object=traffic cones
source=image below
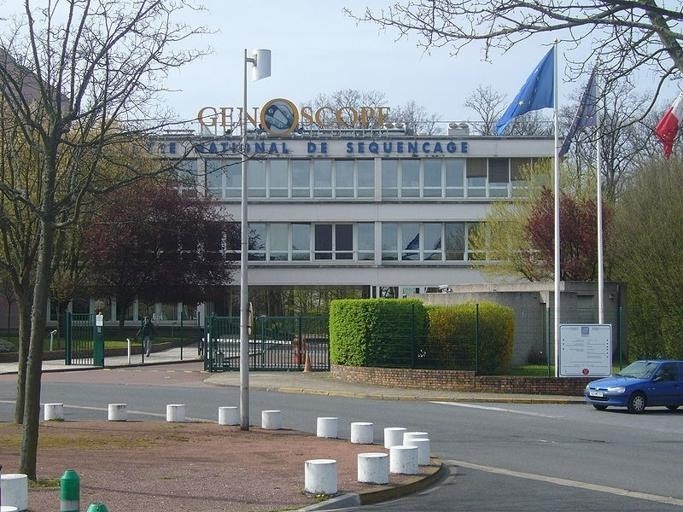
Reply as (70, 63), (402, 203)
(302, 351), (313, 373)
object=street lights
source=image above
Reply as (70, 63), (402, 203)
(240, 48), (272, 430)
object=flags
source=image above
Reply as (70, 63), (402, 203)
(431, 238), (442, 256)
(654, 91), (683, 160)
(493, 44), (555, 135)
(558, 66), (596, 161)
(406, 233), (420, 256)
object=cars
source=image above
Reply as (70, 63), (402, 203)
(585, 359), (683, 414)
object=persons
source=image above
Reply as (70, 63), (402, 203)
(137, 315), (154, 357)
